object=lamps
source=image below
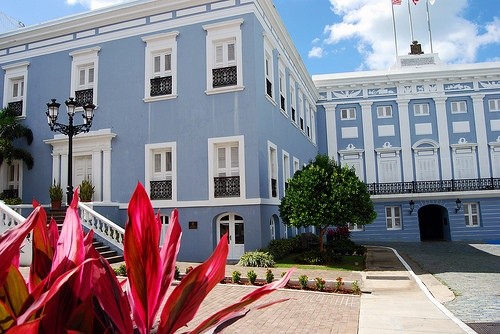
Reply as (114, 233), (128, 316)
(410, 201), (414, 215)
(455, 199), (461, 213)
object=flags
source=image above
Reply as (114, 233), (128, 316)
(412, 0), (421, 5)
(391, 0), (402, 5)
(429, 0), (436, 6)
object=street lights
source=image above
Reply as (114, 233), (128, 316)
(44, 95), (95, 209)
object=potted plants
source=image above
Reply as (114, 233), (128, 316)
(78, 181), (95, 202)
(50, 179), (63, 209)
